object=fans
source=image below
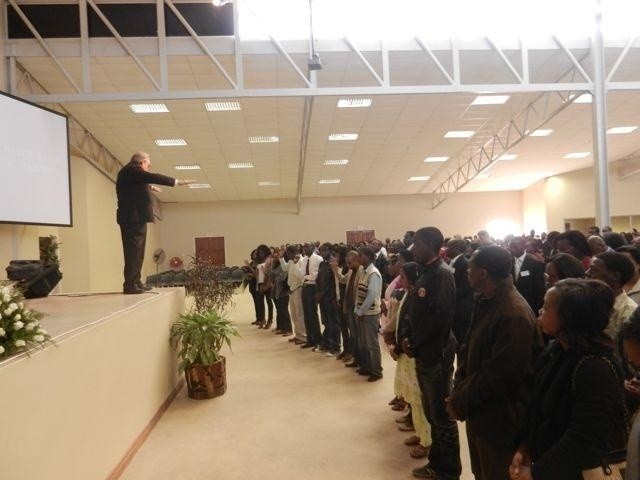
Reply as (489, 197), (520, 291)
(152, 249), (165, 287)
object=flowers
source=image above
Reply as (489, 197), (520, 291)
(0, 279), (60, 359)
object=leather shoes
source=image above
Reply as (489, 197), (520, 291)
(123, 283), (144, 294)
(326, 351), (341, 357)
(138, 280), (153, 291)
(312, 346), (317, 352)
(251, 320), (258, 325)
(283, 332), (293, 337)
(368, 374), (383, 382)
(315, 344), (327, 353)
(359, 367), (371, 375)
(288, 337), (298, 342)
(256, 320), (262, 325)
(346, 360), (360, 367)
(276, 330), (287, 334)
(295, 339), (306, 344)
(301, 343), (313, 350)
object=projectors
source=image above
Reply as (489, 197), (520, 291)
(308, 58), (323, 70)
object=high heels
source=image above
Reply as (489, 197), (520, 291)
(341, 354), (349, 360)
(264, 319), (273, 329)
(258, 319), (267, 329)
(343, 353), (353, 363)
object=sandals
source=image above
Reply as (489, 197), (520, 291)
(391, 397), (407, 411)
(389, 395), (400, 405)
(398, 421), (415, 431)
(410, 445), (427, 459)
(396, 413), (412, 423)
(404, 435), (420, 445)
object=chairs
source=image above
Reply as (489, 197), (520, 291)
(231, 270), (245, 288)
(147, 276), (158, 287)
(216, 270), (230, 289)
(159, 275), (171, 287)
(160, 266), (243, 275)
(171, 274), (188, 288)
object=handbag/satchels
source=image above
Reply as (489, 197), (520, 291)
(572, 355), (631, 480)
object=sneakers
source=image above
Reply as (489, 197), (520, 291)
(413, 462), (438, 477)
(335, 351), (346, 360)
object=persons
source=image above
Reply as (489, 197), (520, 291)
(603, 225), (612, 233)
(605, 231), (625, 247)
(398, 228), (463, 480)
(583, 253), (636, 340)
(279, 245), (307, 346)
(529, 230), (538, 241)
(269, 249), (294, 337)
(551, 230), (595, 270)
(244, 249), (267, 329)
(472, 236), (480, 244)
(539, 233), (547, 245)
(322, 244), (349, 353)
(543, 253), (585, 287)
(620, 232), (634, 240)
(315, 242), (335, 352)
(587, 235), (615, 253)
(445, 238), (472, 339)
(358, 241), (366, 250)
(248, 246), (278, 331)
(477, 230), (496, 246)
(447, 245), (545, 479)
(353, 246), (383, 381)
(295, 242), (324, 348)
(370, 238), (388, 299)
(618, 304), (639, 479)
(618, 242), (640, 261)
(510, 277), (627, 480)
(588, 226), (600, 236)
(386, 262), (432, 459)
(508, 236), (544, 295)
(115, 152), (196, 295)
(330, 250), (364, 367)
(548, 231), (561, 260)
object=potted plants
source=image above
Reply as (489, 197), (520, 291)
(169, 307), (244, 400)
